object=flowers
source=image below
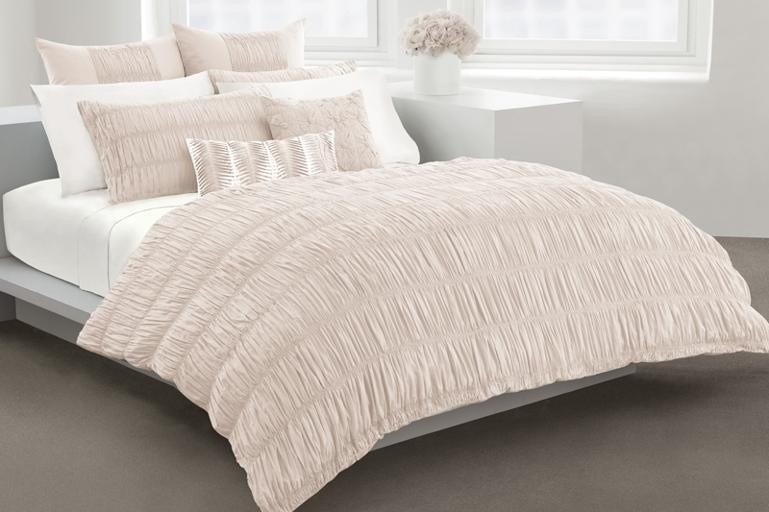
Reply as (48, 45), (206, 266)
(399, 9), (479, 60)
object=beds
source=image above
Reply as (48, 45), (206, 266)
(1, 16), (769, 512)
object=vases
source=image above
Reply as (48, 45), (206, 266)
(414, 52), (464, 97)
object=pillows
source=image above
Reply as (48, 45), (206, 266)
(26, 69), (218, 203)
(213, 68), (422, 175)
(170, 15), (312, 76)
(30, 28), (184, 85)
(183, 129), (342, 200)
(208, 56), (362, 91)
(74, 85), (275, 204)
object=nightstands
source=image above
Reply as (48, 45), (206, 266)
(389, 77), (584, 180)
